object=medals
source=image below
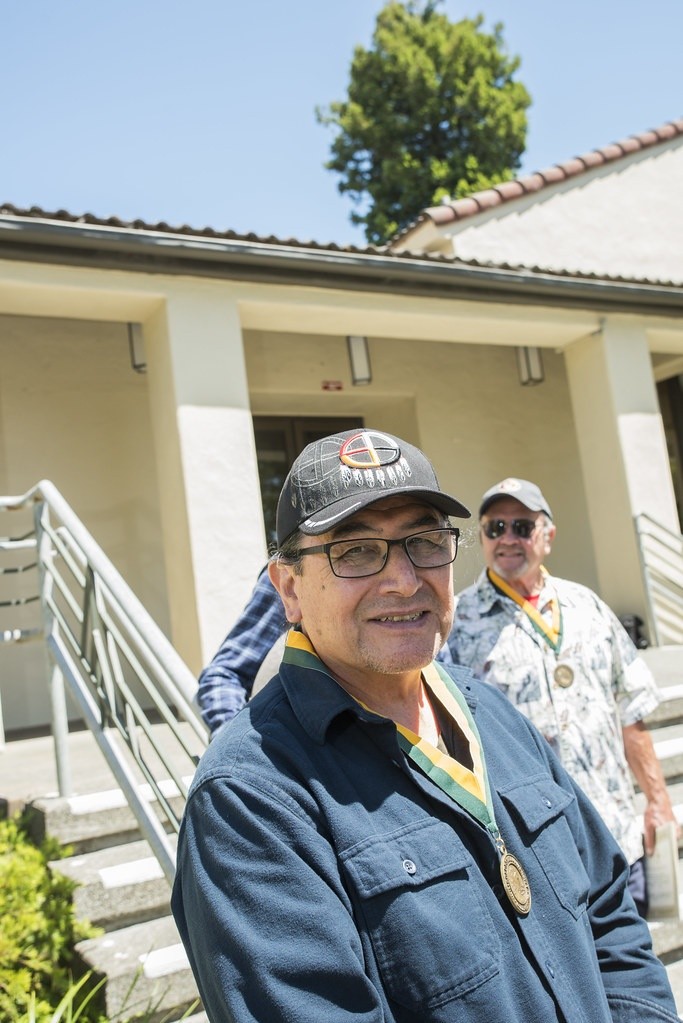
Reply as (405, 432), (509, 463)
(500, 853), (532, 914)
(554, 664), (575, 687)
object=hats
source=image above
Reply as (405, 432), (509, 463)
(276, 428), (471, 550)
(478, 478), (553, 522)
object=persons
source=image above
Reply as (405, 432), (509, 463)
(172, 430), (683, 1021)
(195, 557), (294, 731)
(449, 478), (683, 921)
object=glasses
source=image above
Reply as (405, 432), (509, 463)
(282, 528), (461, 578)
(481, 520), (545, 539)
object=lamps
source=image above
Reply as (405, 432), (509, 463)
(516, 349), (545, 384)
(346, 336), (371, 385)
(128, 322), (147, 373)
